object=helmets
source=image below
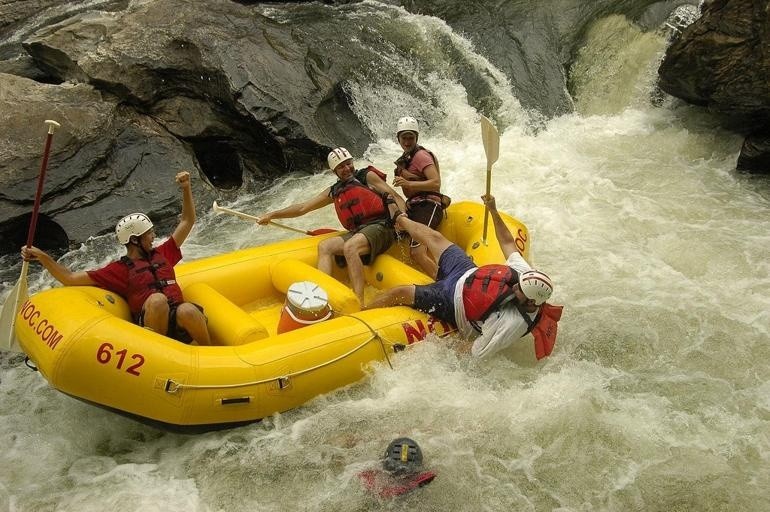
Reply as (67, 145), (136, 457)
(397, 117), (419, 137)
(328, 147), (353, 172)
(520, 269), (553, 306)
(383, 438), (423, 473)
(115, 213), (154, 245)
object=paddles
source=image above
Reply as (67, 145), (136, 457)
(211, 200), (340, 236)
(479, 112), (501, 248)
(0, 118), (62, 354)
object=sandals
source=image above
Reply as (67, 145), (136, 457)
(382, 192), (402, 229)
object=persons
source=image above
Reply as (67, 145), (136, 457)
(20, 170), (212, 348)
(356, 434), (438, 503)
(392, 116), (444, 281)
(256, 147), (410, 315)
(361, 189), (553, 363)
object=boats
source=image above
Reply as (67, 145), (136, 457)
(17, 200), (539, 431)
